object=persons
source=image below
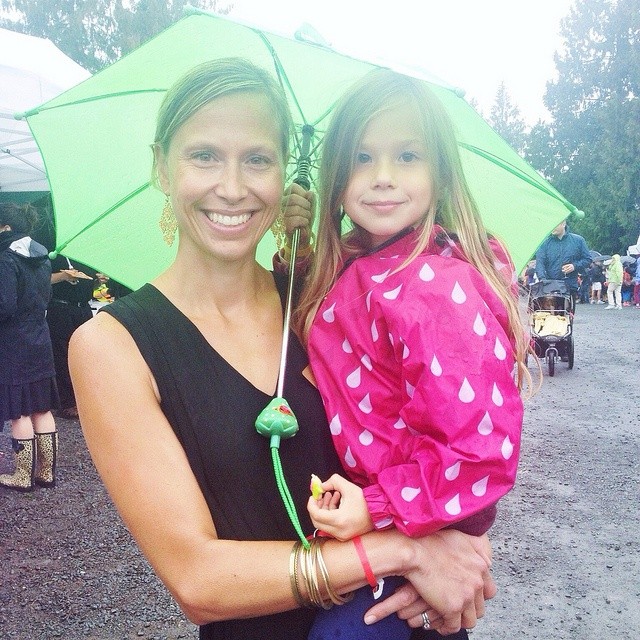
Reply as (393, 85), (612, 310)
(293, 69), (525, 640)
(66, 59), (498, 640)
(590, 248), (639, 309)
(49, 253), (98, 419)
(534, 218), (589, 315)
(1, 201), (59, 491)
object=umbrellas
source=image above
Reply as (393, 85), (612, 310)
(11, 4), (589, 440)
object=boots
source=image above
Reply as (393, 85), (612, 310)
(34, 431), (58, 489)
(1, 438), (36, 490)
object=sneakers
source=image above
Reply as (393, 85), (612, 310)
(603, 304), (614, 311)
(614, 304), (622, 309)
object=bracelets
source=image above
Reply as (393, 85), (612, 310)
(288, 537), (356, 613)
(350, 536), (378, 587)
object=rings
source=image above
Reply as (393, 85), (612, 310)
(421, 612), (433, 628)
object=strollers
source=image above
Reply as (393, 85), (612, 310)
(525, 269), (576, 378)
